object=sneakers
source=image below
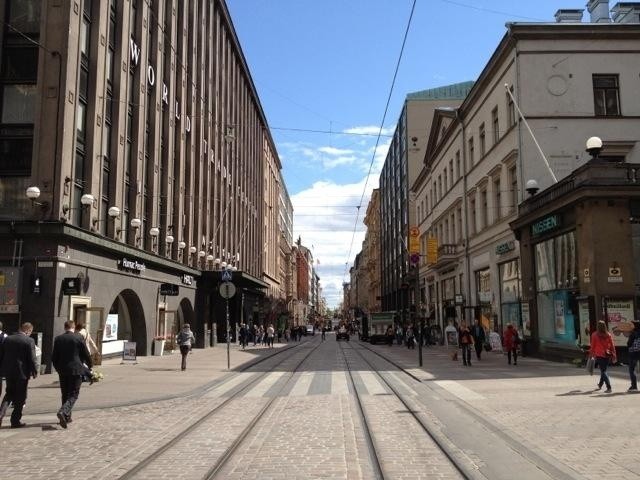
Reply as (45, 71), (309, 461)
(628, 387), (637, 391)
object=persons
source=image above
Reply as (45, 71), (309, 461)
(589, 320), (617, 394)
(1, 320), (99, 429)
(626, 321), (639, 391)
(239, 316), (522, 366)
(176, 325), (196, 370)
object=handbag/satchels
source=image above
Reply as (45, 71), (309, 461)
(511, 335), (521, 343)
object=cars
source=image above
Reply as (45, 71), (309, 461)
(298, 315), (350, 341)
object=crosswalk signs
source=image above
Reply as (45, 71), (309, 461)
(222, 270), (232, 280)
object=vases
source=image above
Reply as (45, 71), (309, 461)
(154, 340), (166, 356)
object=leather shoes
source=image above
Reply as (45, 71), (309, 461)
(11, 423), (25, 428)
(57, 411), (67, 428)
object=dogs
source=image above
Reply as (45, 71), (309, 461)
(448, 350), (459, 361)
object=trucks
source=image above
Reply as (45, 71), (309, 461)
(356, 309), (396, 345)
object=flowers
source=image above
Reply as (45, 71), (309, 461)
(154, 336), (165, 340)
(90, 371), (104, 381)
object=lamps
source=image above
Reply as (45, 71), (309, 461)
(92, 206), (120, 226)
(152, 235), (174, 250)
(223, 123), (238, 145)
(525, 180), (539, 197)
(408, 136), (421, 154)
(116, 218), (141, 235)
(137, 228), (159, 244)
(63, 194), (94, 215)
(585, 136), (604, 159)
(26, 185), (51, 212)
(169, 241), (237, 272)
